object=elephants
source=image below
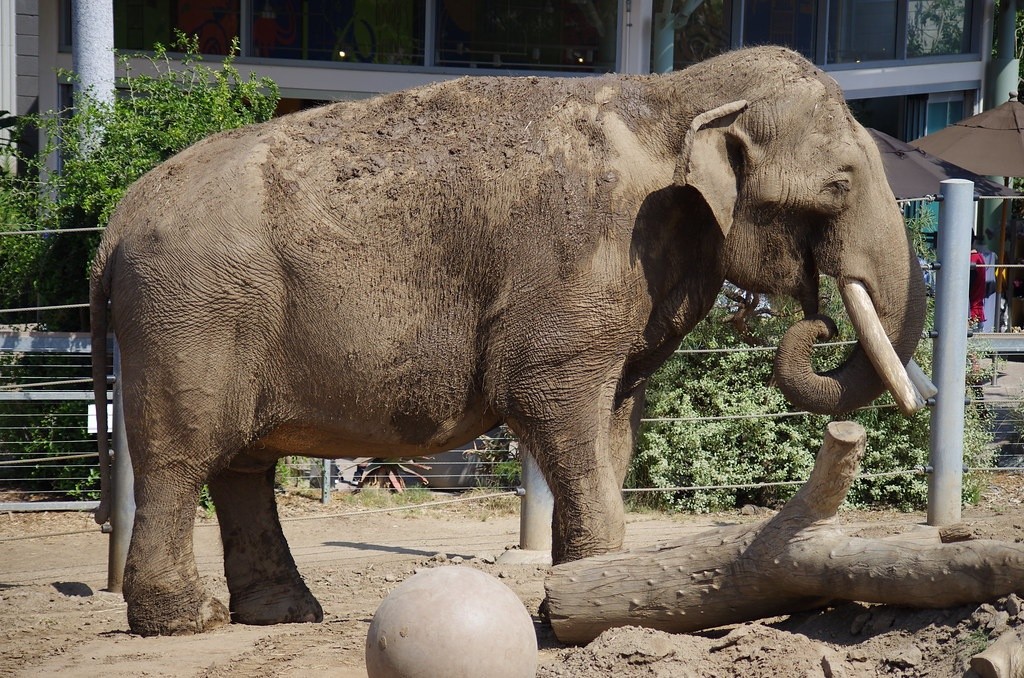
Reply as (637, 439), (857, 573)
(88, 43), (938, 639)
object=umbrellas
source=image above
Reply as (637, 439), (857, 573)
(865, 91), (1024, 388)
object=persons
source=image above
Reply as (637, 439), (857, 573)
(970, 242), (999, 334)
(967, 226), (987, 385)
